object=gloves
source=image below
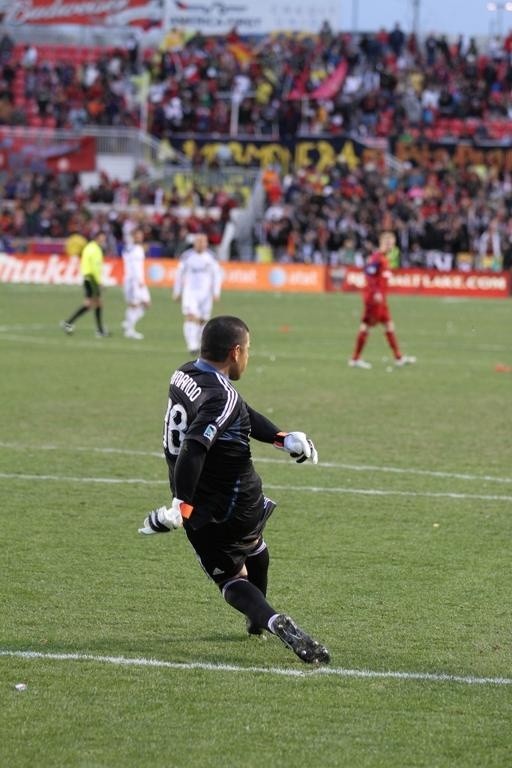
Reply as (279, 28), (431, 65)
(137, 498), (184, 535)
(273, 431), (319, 464)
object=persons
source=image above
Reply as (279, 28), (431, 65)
(58, 231), (110, 336)
(0, 15), (512, 275)
(138, 315), (333, 665)
(120, 230), (151, 341)
(172, 234), (224, 355)
(348, 232), (415, 369)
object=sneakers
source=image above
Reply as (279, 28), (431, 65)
(393, 355), (416, 367)
(267, 613), (331, 667)
(348, 358), (369, 370)
(59, 319), (144, 340)
(245, 618), (264, 638)
